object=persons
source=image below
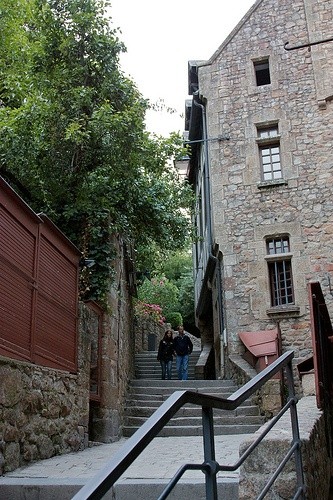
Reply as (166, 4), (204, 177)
(157, 330), (175, 380)
(171, 326), (193, 380)
